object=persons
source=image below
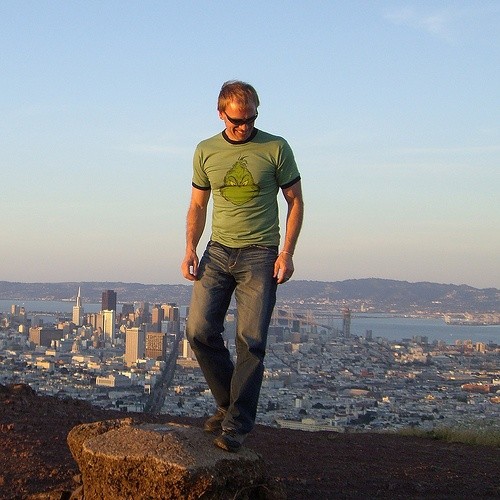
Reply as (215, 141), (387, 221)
(182, 80), (305, 454)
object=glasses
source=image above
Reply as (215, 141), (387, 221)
(224, 111), (258, 125)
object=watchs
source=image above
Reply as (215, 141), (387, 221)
(282, 250), (293, 257)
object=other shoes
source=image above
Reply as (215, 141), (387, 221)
(203, 407), (228, 433)
(214, 425), (250, 451)
